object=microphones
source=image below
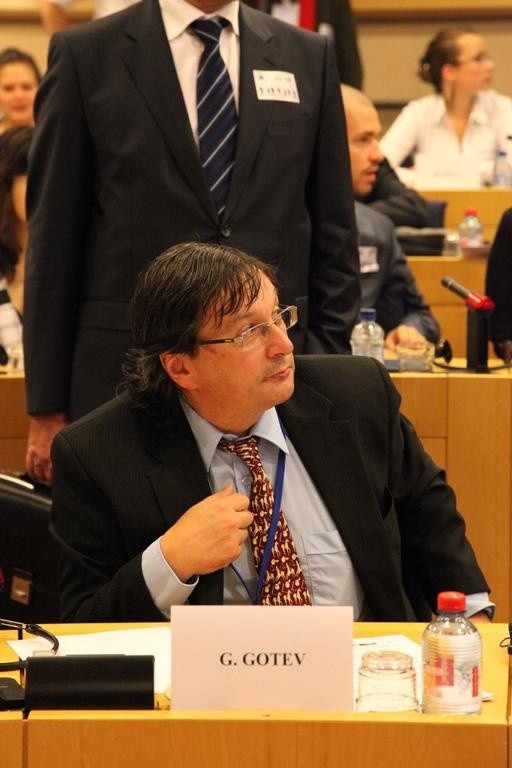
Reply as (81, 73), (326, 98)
(441, 276), (493, 311)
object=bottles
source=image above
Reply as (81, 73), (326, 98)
(460, 209), (483, 248)
(349, 307), (383, 367)
(422, 592), (483, 718)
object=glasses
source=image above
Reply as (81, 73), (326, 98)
(195, 305), (298, 351)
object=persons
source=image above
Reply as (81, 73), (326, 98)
(0, 48), (43, 373)
(23, 0), (362, 488)
(28, 240), (499, 625)
(486, 208), (511, 369)
(340, 82), (454, 365)
(372, 27), (510, 189)
(246, 0), (362, 92)
(42, 2), (136, 32)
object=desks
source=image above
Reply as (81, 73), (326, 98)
(2, 618), (511, 768)
(1, 357), (512, 624)
(0, 185), (510, 358)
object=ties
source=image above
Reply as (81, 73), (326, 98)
(190, 16), (239, 218)
(219, 435), (308, 606)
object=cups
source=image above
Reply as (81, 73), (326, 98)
(356, 650), (420, 712)
(396, 342), (436, 374)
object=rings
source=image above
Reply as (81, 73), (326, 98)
(32, 462), (45, 470)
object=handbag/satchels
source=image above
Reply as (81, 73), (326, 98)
(0, 472), (71, 628)
